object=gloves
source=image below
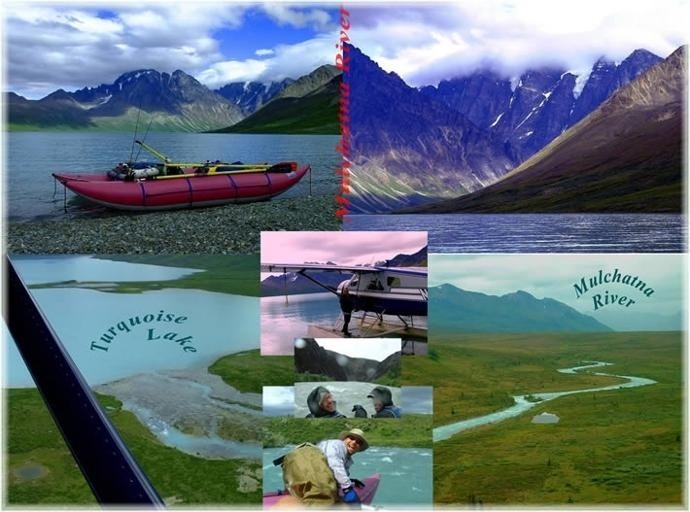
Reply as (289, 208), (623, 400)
(343, 488), (361, 504)
(350, 479), (364, 488)
(340, 429), (368, 451)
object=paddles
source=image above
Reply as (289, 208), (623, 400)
(152, 161), (297, 179)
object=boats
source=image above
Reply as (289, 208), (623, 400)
(263, 472), (382, 509)
(50, 160), (312, 212)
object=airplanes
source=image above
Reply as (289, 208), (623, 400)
(260, 262), (428, 356)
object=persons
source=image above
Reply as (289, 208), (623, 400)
(336, 273), (359, 336)
(274, 428), (369, 509)
(305, 386), (401, 419)
